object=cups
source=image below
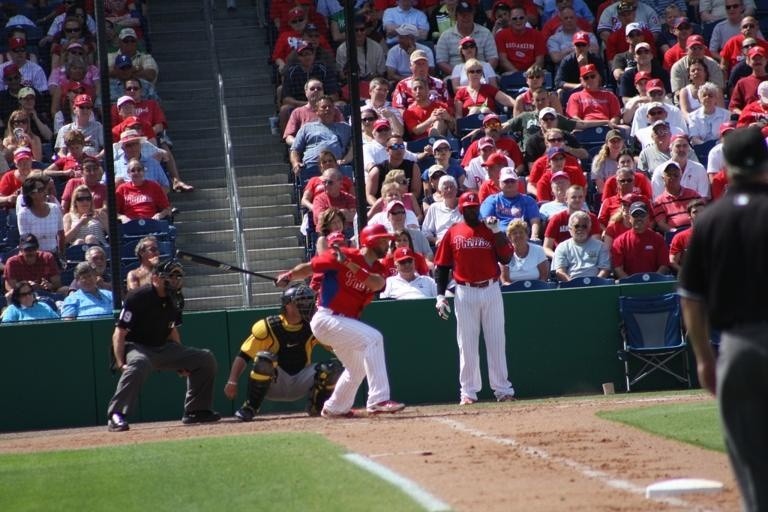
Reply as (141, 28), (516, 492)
(602, 383), (614, 394)
(13, 127), (26, 147)
(269, 117), (280, 136)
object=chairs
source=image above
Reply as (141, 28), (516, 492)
(618, 293), (692, 390)
(0, 1), (186, 316)
(294, 9), (727, 292)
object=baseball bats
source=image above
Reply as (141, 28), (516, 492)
(176, 248), (290, 286)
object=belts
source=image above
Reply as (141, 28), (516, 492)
(457, 278), (497, 287)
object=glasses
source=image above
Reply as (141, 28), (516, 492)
(10, 26), (143, 298)
(321, 180), (339, 185)
(511, 16), (526, 21)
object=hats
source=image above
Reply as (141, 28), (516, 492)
(456, 2), (473, 13)
(288, 7), (304, 22)
(395, 247), (413, 261)
(304, 24), (318, 34)
(617, 1), (636, 13)
(371, 32), (768, 216)
(395, 23), (419, 36)
(674, 17), (690, 29)
(296, 42), (313, 53)
(625, 23), (643, 38)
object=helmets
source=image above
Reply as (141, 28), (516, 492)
(282, 283), (315, 322)
(360, 225), (397, 251)
(155, 261), (185, 294)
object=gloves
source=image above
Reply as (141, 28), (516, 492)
(273, 273), (292, 286)
(436, 295), (452, 320)
(328, 244), (346, 263)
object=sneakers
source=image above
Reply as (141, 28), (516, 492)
(308, 402), (325, 417)
(365, 400), (405, 415)
(459, 397), (474, 407)
(107, 413), (128, 432)
(321, 405), (363, 417)
(235, 400), (260, 422)
(496, 393), (514, 402)
(182, 411), (222, 424)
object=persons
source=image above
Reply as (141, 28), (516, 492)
(677, 127), (766, 512)
(431, 188), (518, 407)
(228, 2), (768, 306)
(1, 1), (194, 322)
(224, 285), (343, 420)
(269, 223), (408, 420)
(105, 260), (220, 433)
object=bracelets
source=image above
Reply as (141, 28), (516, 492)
(227, 381), (239, 387)
